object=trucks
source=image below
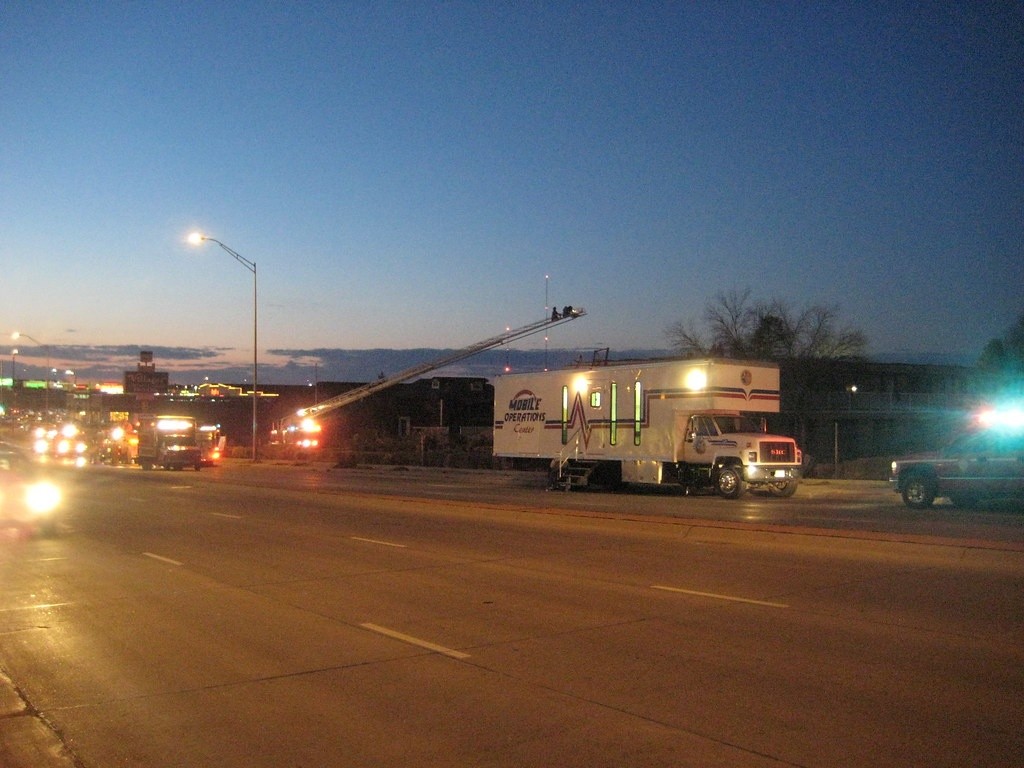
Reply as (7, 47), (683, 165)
(135, 413), (203, 473)
(494, 357), (806, 499)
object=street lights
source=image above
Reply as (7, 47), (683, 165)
(187, 234), (258, 466)
(12, 333), (49, 423)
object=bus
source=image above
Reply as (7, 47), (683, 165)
(197, 425), (222, 467)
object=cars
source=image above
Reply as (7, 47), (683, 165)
(888, 421), (1023, 510)
